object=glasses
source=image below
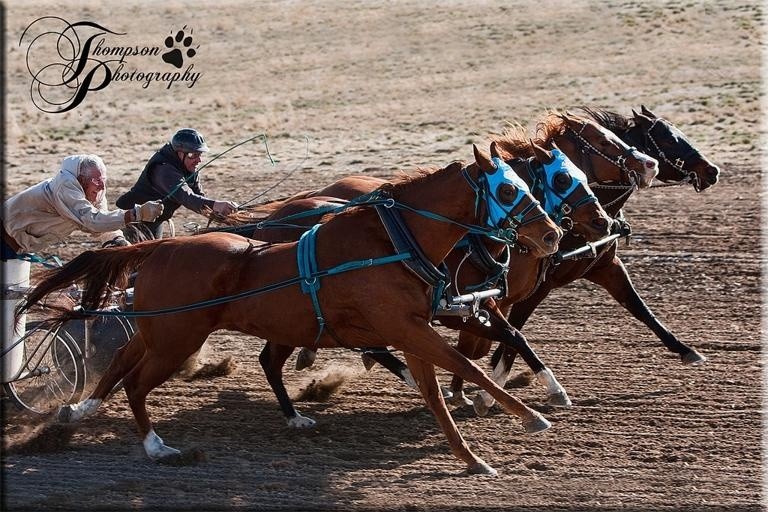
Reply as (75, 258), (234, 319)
(188, 152), (200, 159)
(79, 175), (106, 186)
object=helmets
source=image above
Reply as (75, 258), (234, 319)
(170, 128), (209, 152)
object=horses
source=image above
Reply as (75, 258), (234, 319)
(13, 101), (723, 478)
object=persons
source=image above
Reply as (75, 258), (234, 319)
(116, 128), (238, 239)
(3, 154), (164, 259)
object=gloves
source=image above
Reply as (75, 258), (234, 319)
(134, 199), (164, 223)
